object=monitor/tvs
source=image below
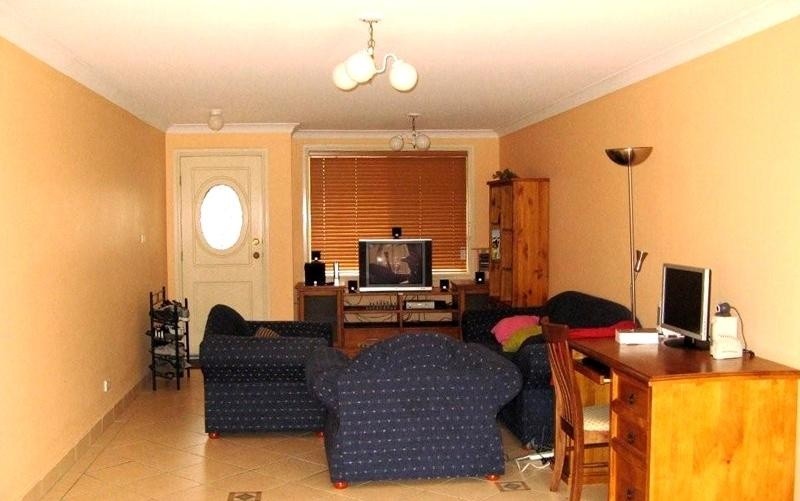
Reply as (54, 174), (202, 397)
(661, 264), (711, 341)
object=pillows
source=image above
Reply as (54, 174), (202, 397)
(253, 326), (280, 337)
(490, 313), (539, 341)
(501, 324), (543, 351)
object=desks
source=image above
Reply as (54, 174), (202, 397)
(550, 329), (800, 500)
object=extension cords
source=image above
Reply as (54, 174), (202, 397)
(528, 451), (555, 460)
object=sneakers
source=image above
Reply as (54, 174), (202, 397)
(149, 300), (190, 323)
(149, 343), (187, 357)
(146, 324), (184, 341)
(149, 359), (192, 378)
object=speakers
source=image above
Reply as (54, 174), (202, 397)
(440, 279), (449, 292)
(392, 227), (402, 239)
(311, 250), (320, 263)
(475, 272), (485, 284)
(304, 263), (325, 286)
(348, 280), (357, 293)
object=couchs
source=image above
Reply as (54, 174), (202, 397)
(461, 288), (643, 449)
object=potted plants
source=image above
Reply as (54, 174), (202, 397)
(491, 167), (519, 180)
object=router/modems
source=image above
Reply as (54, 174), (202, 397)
(661, 326), (685, 339)
(406, 300), (435, 309)
(614, 328), (658, 345)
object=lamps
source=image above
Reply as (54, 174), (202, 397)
(605, 144), (657, 344)
(388, 111), (431, 152)
(331, 13), (418, 92)
(206, 108), (224, 131)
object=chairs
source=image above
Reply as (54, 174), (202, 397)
(307, 330), (527, 489)
(539, 322), (610, 500)
(198, 301), (333, 439)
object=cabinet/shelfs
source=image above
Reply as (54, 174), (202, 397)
(293, 280), (488, 358)
(148, 285), (191, 392)
(486, 177), (550, 307)
